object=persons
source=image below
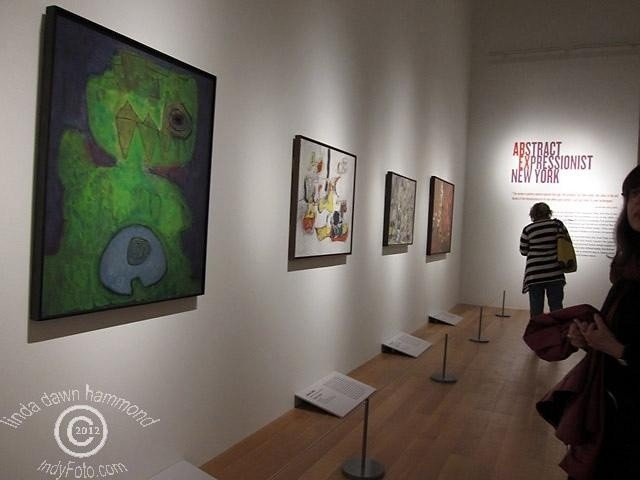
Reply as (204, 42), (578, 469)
(567, 166), (639, 479)
(519, 202), (572, 318)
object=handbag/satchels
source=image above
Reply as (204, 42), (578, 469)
(556, 218), (577, 273)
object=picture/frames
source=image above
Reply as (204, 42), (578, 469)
(382, 169), (417, 246)
(29, 2), (218, 326)
(289, 133), (357, 261)
(424, 174), (456, 257)
